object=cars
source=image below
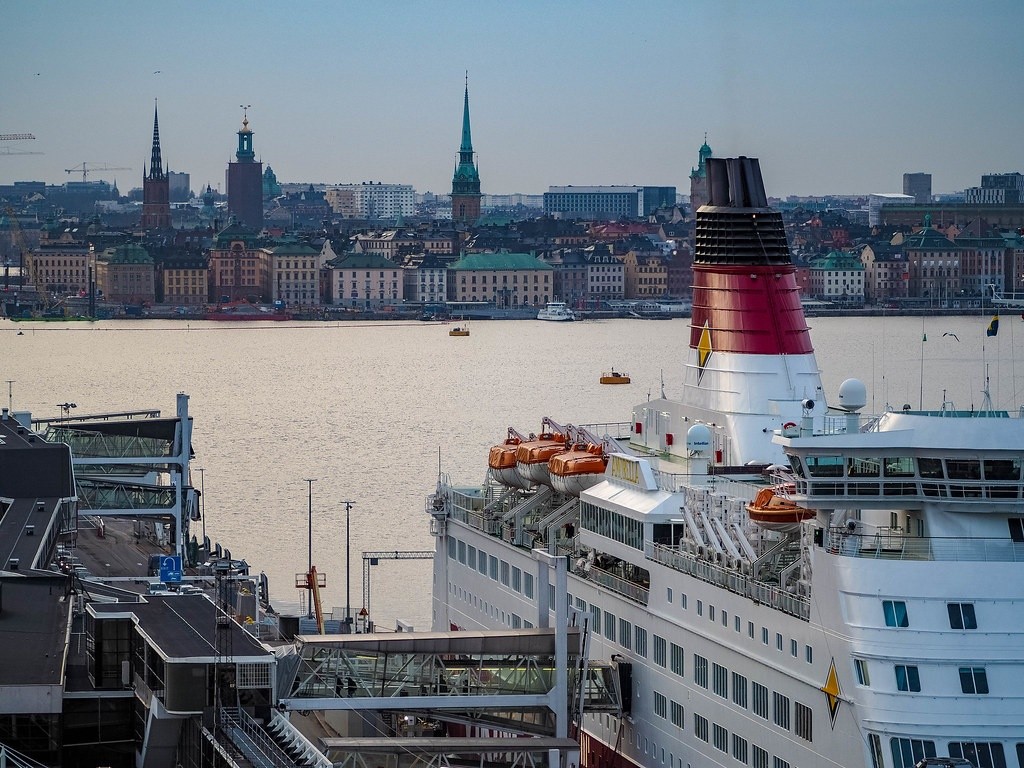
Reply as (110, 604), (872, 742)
(184, 588), (206, 595)
(824, 302), (899, 310)
(176, 584), (193, 595)
(156, 592), (178, 595)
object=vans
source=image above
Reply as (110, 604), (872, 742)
(145, 581), (167, 594)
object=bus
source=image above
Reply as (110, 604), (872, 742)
(147, 553), (165, 577)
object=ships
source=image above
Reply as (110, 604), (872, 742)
(536, 296), (575, 322)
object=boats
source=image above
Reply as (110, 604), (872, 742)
(420, 311), (446, 321)
(600, 367), (630, 384)
(448, 325), (469, 336)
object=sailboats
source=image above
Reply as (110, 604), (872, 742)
(987, 309), (999, 337)
(1021, 313), (1024, 322)
